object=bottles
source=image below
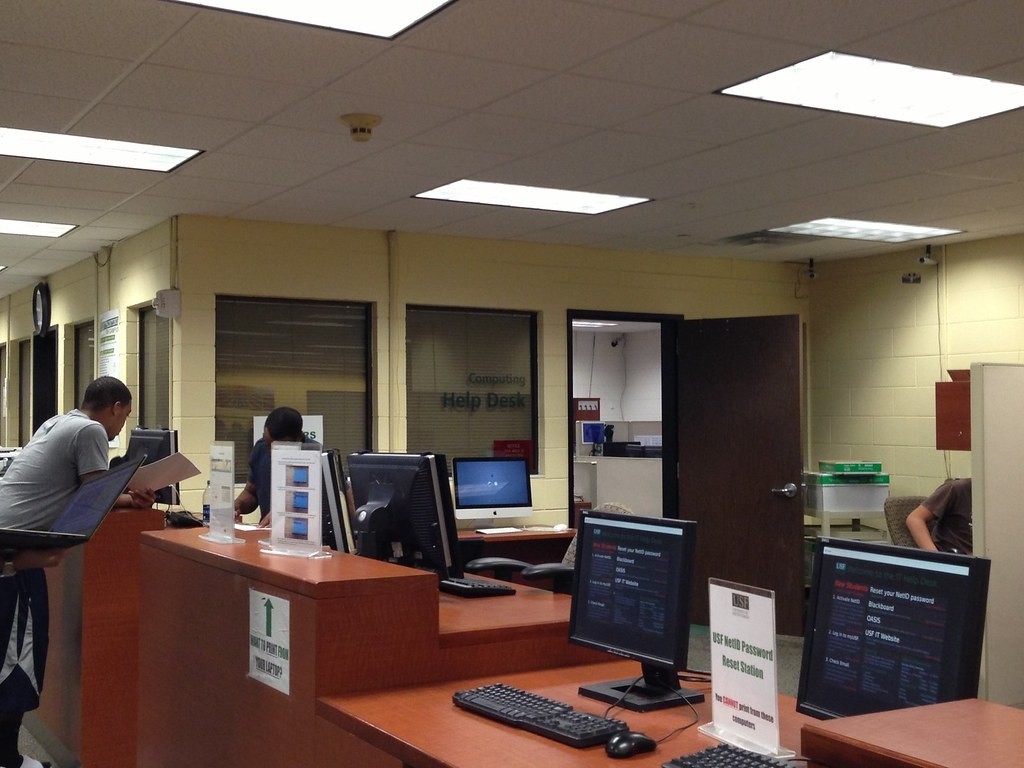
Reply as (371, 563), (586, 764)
(203, 480), (210, 527)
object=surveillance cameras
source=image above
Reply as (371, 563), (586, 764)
(808, 272), (816, 280)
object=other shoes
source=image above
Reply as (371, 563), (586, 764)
(19, 753), (53, 768)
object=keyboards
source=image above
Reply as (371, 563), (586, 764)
(661, 744), (807, 768)
(438, 576), (517, 597)
(452, 682), (630, 748)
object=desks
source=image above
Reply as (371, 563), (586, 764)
(458, 527), (578, 592)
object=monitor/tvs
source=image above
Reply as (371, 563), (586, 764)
(319, 449), (353, 554)
(625, 445), (663, 458)
(450, 456), (532, 531)
(110, 428), (181, 507)
(348, 450), (464, 584)
(581, 422), (604, 445)
(567, 508), (705, 714)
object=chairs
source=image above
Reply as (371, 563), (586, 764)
(465, 504), (634, 595)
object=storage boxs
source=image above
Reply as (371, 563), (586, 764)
(804, 461), (890, 511)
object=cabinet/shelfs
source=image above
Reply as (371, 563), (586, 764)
(804, 507), (891, 613)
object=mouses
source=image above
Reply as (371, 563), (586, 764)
(604, 730), (657, 759)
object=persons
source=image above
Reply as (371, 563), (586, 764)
(0, 376), (156, 768)
(234, 407), (324, 528)
(906, 478), (972, 555)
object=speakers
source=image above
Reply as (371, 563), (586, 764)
(796, 535), (992, 720)
